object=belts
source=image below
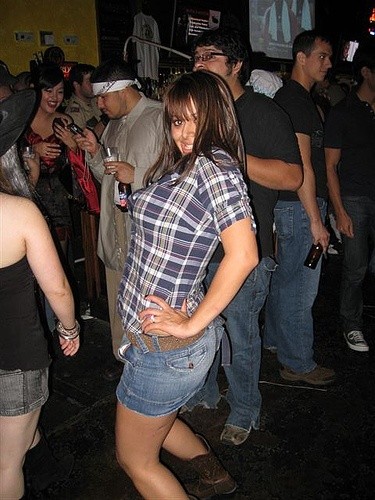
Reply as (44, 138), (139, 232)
(127, 327), (206, 351)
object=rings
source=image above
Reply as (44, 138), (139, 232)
(150, 314), (155, 323)
(46, 152), (50, 156)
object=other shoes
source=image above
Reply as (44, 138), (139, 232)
(102, 361), (124, 381)
(49, 336), (71, 378)
(78, 319), (105, 351)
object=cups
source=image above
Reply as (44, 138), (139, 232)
(100, 146), (119, 174)
(21, 145), (36, 170)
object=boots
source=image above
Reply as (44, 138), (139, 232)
(24, 425), (75, 492)
(182, 434), (238, 500)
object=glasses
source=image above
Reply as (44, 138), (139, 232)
(191, 51), (227, 64)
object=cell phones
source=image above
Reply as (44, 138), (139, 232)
(70, 125), (86, 137)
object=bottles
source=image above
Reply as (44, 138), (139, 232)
(118, 183), (132, 213)
(304, 221), (331, 270)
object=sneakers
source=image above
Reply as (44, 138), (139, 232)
(343, 331), (369, 351)
(220, 425), (250, 446)
(279, 364), (337, 385)
(178, 403), (203, 414)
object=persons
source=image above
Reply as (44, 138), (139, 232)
(71, 29), (375, 385)
(180, 28), (305, 448)
(116, 69), (258, 500)
(251, 0), (314, 56)
(0, 87), (80, 500)
(0, 59), (109, 335)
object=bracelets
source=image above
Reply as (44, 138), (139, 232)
(57, 319), (80, 341)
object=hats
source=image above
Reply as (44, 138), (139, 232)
(0, 88), (43, 157)
(0, 60), (18, 84)
